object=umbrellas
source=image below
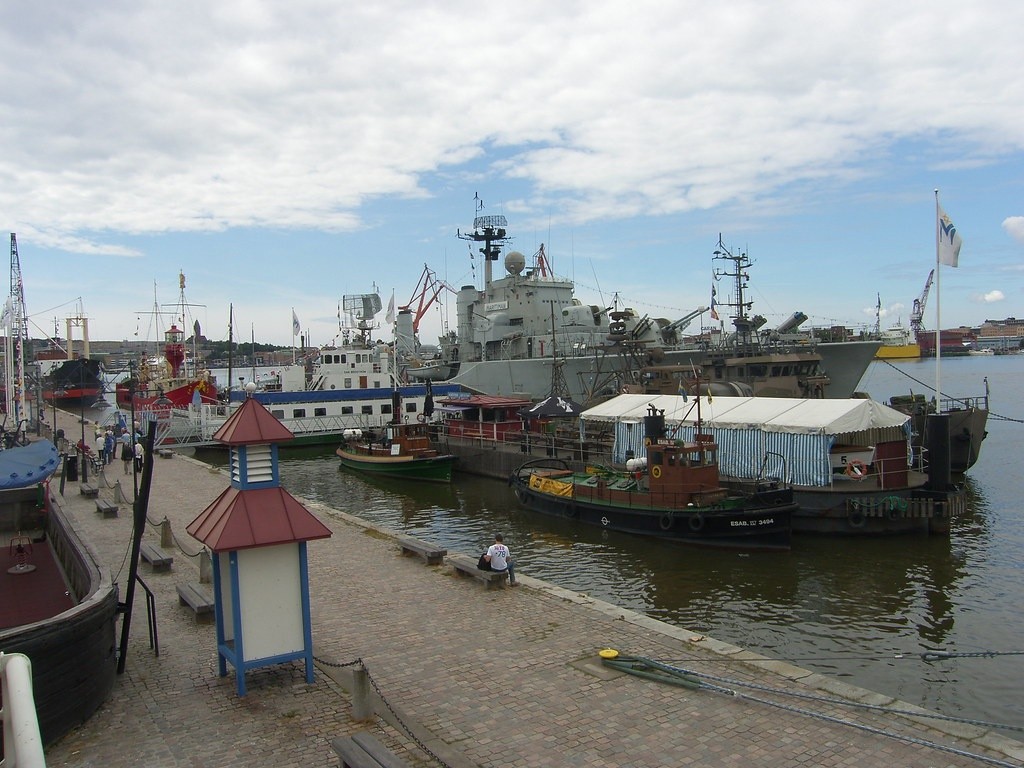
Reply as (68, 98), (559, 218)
(517, 394), (590, 446)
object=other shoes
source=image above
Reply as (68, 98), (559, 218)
(136, 470), (141, 473)
(124, 472), (132, 475)
(511, 581), (521, 586)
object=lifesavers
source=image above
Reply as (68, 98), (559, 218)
(847, 460), (867, 479)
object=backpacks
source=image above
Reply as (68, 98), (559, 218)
(477, 553), (491, 571)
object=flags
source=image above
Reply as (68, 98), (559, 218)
(385, 293), (394, 325)
(937, 200), (962, 268)
(712, 298), (717, 307)
(711, 308), (720, 321)
(679, 382), (688, 404)
(293, 310), (300, 336)
(284, 364), (289, 372)
(712, 285), (716, 297)
(271, 370), (275, 376)
(707, 385), (713, 404)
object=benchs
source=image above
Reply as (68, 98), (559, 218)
(398, 537), (447, 566)
(159, 450), (175, 459)
(175, 581), (216, 624)
(95, 499), (119, 519)
(140, 543), (174, 574)
(80, 482), (99, 498)
(450, 556), (508, 589)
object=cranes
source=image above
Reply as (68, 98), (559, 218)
(0, 229), (47, 432)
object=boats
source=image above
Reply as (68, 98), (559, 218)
(166, 179), (1001, 550)
(37, 294), (105, 409)
(0, 433), (129, 765)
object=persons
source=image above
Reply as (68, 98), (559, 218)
(40, 408), (45, 420)
(484, 534), (520, 588)
(635, 467), (644, 492)
(77, 439), (89, 451)
(94, 417), (144, 475)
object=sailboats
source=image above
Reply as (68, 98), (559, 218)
(117, 267), (223, 426)
